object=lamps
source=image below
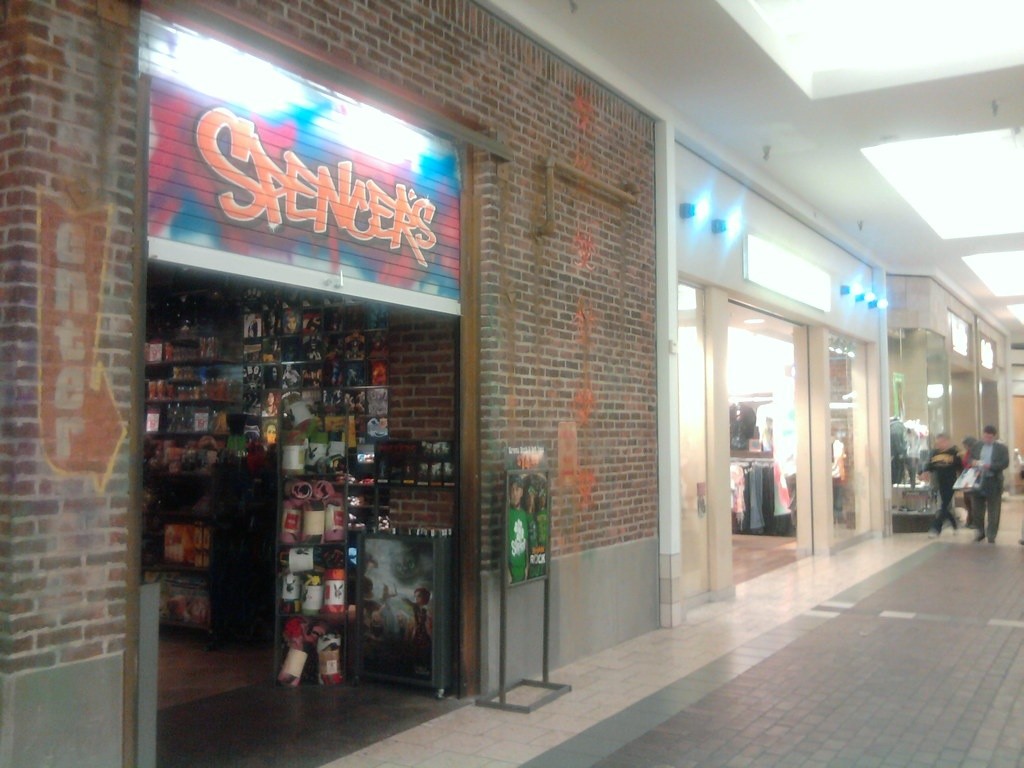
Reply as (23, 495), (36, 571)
(712, 219), (727, 234)
(855, 292), (866, 302)
(840, 285), (850, 296)
(868, 299), (878, 309)
(679, 202), (697, 219)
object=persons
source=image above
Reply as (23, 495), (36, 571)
(961, 436), (975, 530)
(968, 425), (1009, 543)
(832, 439), (845, 524)
(904, 420), (919, 487)
(918, 424), (929, 475)
(924, 433), (963, 537)
(509, 477), (549, 583)
(891, 417), (908, 484)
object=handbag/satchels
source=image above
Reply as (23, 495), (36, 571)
(952, 463), (984, 492)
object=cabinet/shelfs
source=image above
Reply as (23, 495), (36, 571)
(143, 358), (239, 442)
(273, 397), (381, 692)
(143, 463), (272, 653)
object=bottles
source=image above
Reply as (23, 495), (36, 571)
(164, 337), (219, 360)
(149, 379), (234, 400)
(205, 406), (217, 430)
(161, 402), (193, 431)
(173, 365), (208, 380)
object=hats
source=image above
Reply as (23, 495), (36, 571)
(525, 485), (536, 495)
(538, 488), (547, 499)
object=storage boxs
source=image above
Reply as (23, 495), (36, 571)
(163, 523), (212, 568)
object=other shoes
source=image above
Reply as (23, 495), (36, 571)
(927, 526), (939, 539)
(987, 535), (996, 543)
(976, 534), (985, 542)
(969, 524), (979, 529)
